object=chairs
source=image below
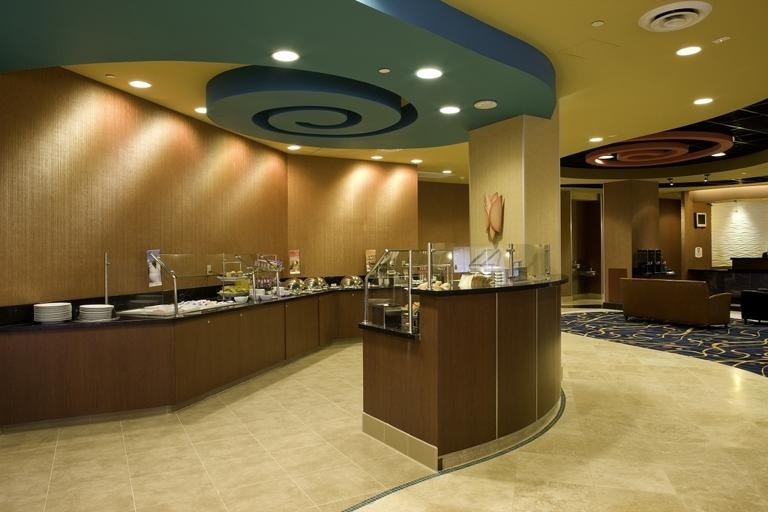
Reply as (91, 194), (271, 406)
(740, 286), (768, 326)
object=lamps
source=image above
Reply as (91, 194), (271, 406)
(666, 177), (674, 187)
(702, 173), (712, 183)
(483, 191), (504, 243)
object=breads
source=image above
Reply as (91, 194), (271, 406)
(458, 274), (492, 290)
(418, 279), (450, 291)
(404, 302), (420, 312)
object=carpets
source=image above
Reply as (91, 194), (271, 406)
(557, 308), (768, 379)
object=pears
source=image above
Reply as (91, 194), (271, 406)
(226, 271), (243, 277)
(221, 286), (248, 293)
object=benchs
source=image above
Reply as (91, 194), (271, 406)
(618, 276), (734, 333)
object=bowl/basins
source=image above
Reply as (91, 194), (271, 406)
(259, 295), (273, 301)
(217, 291), (246, 298)
(234, 296), (249, 303)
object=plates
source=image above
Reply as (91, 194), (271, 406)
(249, 289), (265, 300)
(306, 288), (322, 291)
(273, 287), (290, 297)
(77, 303), (115, 323)
(33, 302), (72, 323)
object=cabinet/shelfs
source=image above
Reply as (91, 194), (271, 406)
(165, 284), (421, 415)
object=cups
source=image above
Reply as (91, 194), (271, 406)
(225, 272), (244, 278)
